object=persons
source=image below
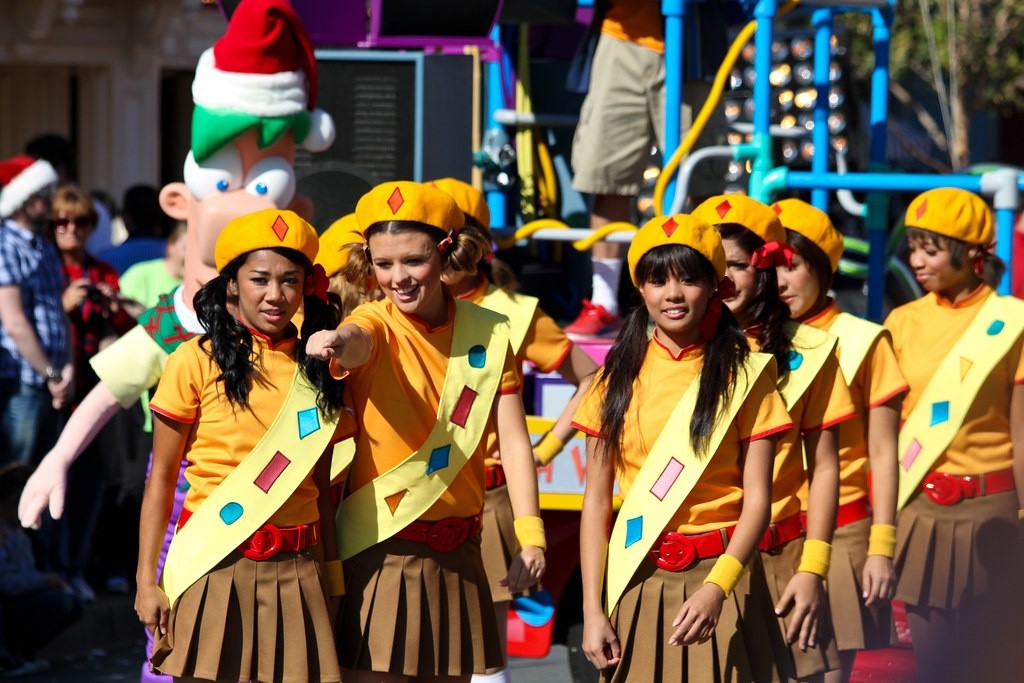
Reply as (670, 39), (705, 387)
(569, 190), (912, 682)
(563, 1), (728, 343)
(879, 188), (1022, 682)
(0, 153), (189, 677)
(134, 176), (601, 683)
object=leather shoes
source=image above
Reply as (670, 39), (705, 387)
(758, 511), (803, 551)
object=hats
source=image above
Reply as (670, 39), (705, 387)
(215, 209), (319, 272)
(313, 211), (365, 278)
(770, 198), (844, 273)
(628, 214), (726, 289)
(904, 187), (994, 244)
(690, 195), (786, 251)
(425, 177), (490, 231)
(191, 0), (336, 153)
(1, 156), (58, 220)
(355, 180), (465, 240)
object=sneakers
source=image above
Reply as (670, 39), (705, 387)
(561, 299), (626, 341)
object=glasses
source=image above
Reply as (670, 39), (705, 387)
(55, 217), (92, 229)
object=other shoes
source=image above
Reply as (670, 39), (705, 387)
(72, 578), (95, 604)
(107, 580), (131, 597)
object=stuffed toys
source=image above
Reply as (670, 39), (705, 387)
(13, 0), (336, 682)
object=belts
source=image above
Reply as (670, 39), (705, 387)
(919, 468), (1015, 505)
(647, 525), (736, 571)
(177, 506), (320, 560)
(396, 502), (485, 553)
(835, 494), (871, 527)
